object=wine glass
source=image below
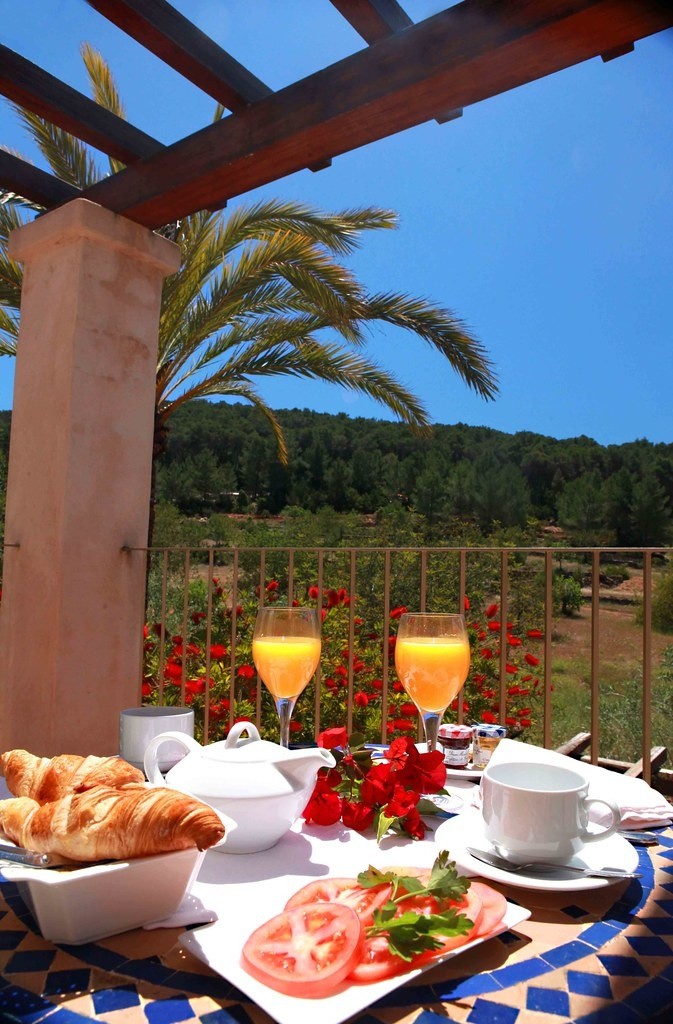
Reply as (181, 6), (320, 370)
(251, 606), (322, 749)
(394, 612), (471, 753)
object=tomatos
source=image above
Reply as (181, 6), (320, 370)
(241, 866), (507, 992)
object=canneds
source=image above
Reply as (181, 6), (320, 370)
(435, 724), (473, 770)
(471, 723), (507, 771)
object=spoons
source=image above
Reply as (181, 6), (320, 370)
(465, 847), (643, 879)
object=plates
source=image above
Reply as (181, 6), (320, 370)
(434, 811), (640, 894)
(445, 766), (483, 781)
(177, 850), (532, 1024)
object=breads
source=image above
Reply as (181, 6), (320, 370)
(0, 786), (225, 861)
(0, 748), (144, 805)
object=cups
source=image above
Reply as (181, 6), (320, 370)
(483, 760), (621, 873)
(472, 724), (507, 769)
(118, 708), (195, 773)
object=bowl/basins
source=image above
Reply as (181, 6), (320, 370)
(0, 791), (239, 946)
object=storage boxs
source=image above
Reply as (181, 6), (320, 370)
(0, 774), (238, 946)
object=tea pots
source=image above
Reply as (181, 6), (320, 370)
(143, 721), (337, 855)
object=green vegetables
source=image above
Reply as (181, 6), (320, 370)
(356, 848), (475, 963)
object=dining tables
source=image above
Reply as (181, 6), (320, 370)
(0, 744), (673, 1024)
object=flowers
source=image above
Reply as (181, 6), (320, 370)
(301, 726), (450, 844)
